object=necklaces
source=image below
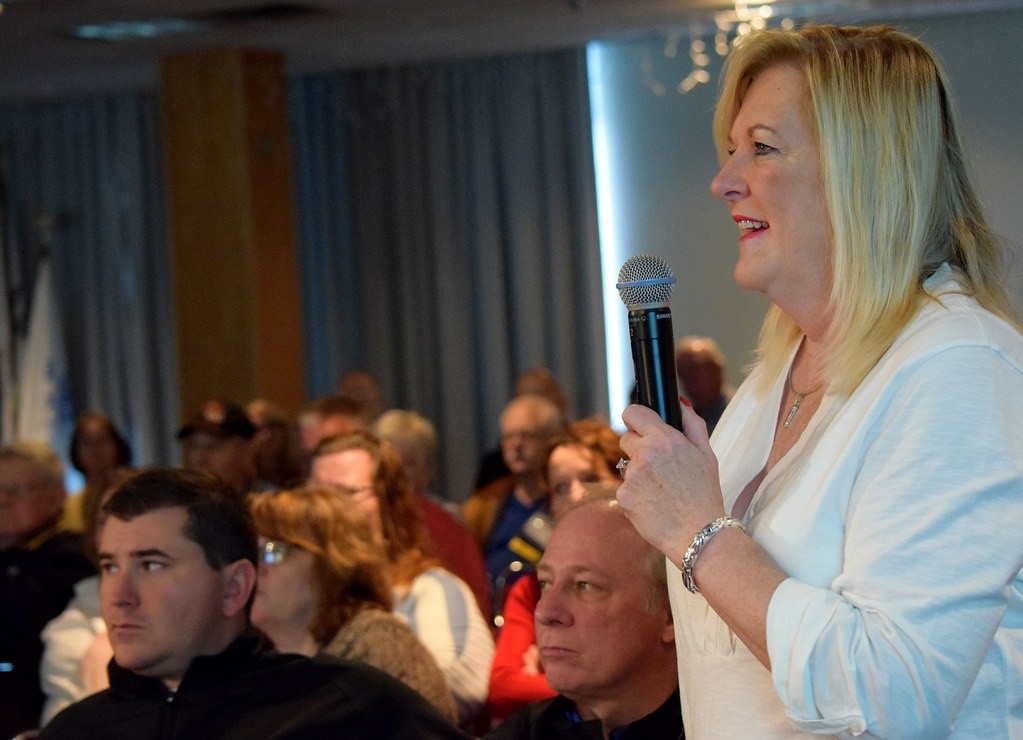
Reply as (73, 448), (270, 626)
(782, 363), (828, 428)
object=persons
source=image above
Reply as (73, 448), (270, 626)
(614, 18), (1023, 738)
(1, 360), (688, 740)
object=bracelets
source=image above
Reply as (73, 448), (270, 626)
(681, 511), (747, 592)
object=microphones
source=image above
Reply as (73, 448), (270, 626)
(615, 255), (685, 434)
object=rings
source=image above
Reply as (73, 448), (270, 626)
(615, 459), (631, 478)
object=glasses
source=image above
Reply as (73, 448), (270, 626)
(255, 539), (304, 566)
(331, 484), (374, 496)
